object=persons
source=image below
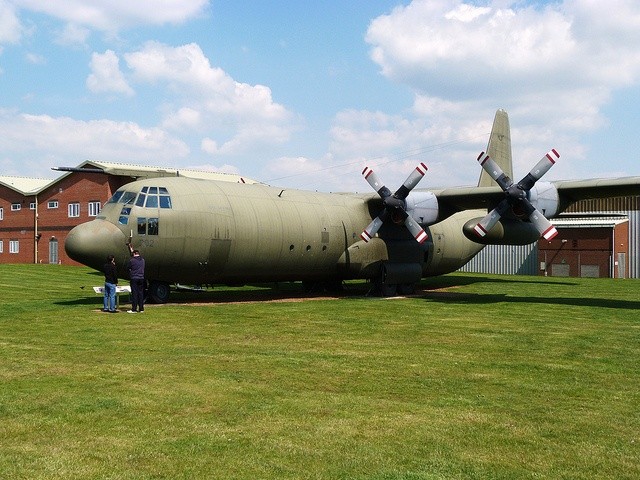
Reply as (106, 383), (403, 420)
(103, 255), (120, 313)
(126, 249), (145, 314)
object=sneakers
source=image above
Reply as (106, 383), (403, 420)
(126, 310), (137, 314)
(109, 310), (120, 312)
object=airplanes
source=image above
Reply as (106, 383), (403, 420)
(51, 109), (561, 304)
